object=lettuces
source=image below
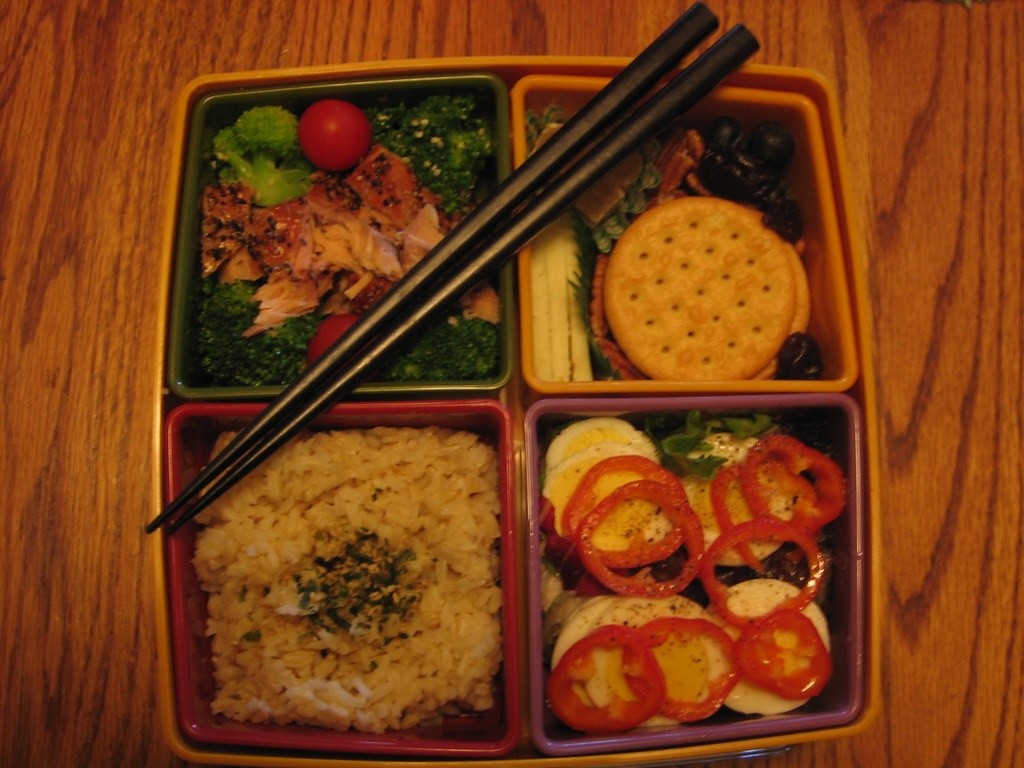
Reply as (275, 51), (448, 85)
(528, 100), (775, 479)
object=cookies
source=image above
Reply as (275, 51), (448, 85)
(601, 195), (811, 379)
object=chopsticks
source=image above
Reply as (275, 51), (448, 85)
(142, 2), (762, 535)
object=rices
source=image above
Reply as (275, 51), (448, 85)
(186, 427), (503, 734)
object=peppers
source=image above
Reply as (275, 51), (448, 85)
(544, 432), (848, 734)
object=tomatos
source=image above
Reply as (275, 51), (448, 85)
(298, 100), (370, 172)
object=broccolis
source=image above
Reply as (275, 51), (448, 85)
(194, 91), (502, 382)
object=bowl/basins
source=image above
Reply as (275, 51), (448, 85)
(524, 393), (865, 758)
(509, 74), (861, 408)
(161, 402), (524, 758)
(166, 68), (516, 402)
